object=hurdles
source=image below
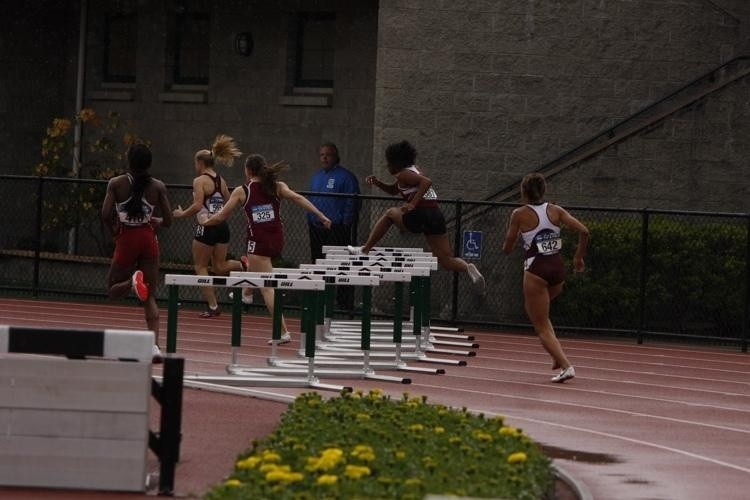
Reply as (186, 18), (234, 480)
(297, 263), (467, 366)
(0, 324), (185, 494)
(321, 245), (476, 341)
(153, 274), (354, 403)
(226, 270), (412, 393)
(314, 259), (477, 357)
(268, 267), (446, 376)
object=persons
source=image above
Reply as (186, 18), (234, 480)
(199, 151), (334, 345)
(502, 171), (592, 382)
(346, 139), (487, 290)
(305, 141), (361, 319)
(100, 143), (173, 365)
(170, 134), (247, 319)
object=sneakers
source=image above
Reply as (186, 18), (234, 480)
(229, 290), (253, 304)
(348, 245), (368, 259)
(240, 255), (249, 272)
(267, 332), (291, 345)
(132, 270), (149, 302)
(199, 305), (221, 318)
(467, 263), (485, 292)
(552, 366), (576, 382)
(153, 344), (162, 355)
